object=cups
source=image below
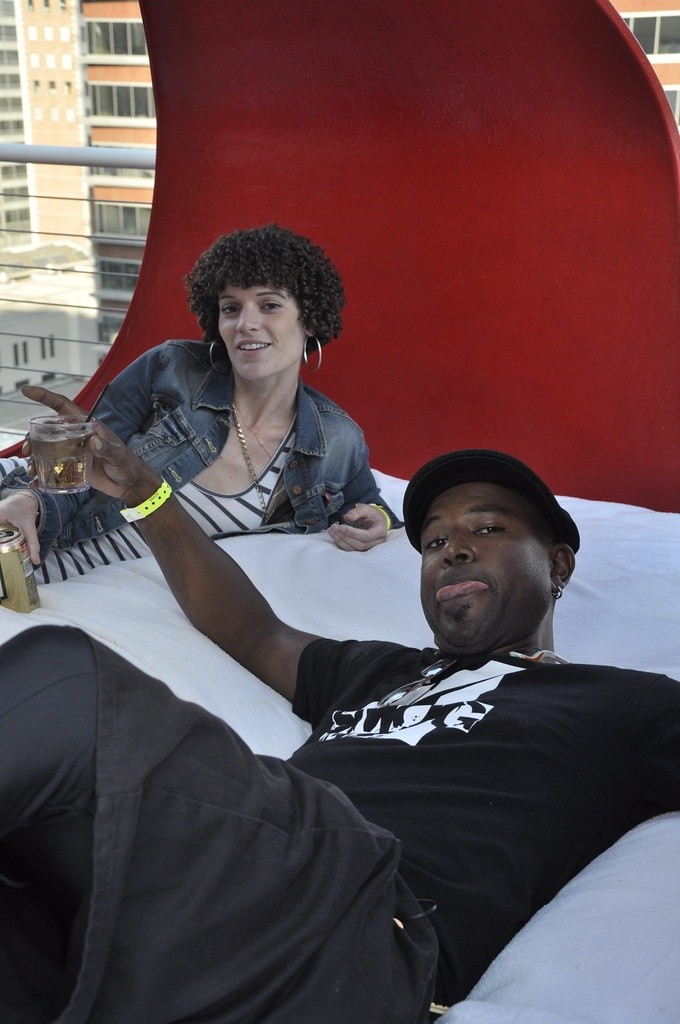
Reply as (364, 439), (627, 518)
(30, 416), (96, 495)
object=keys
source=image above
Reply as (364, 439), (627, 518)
(429, 1001), (448, 1014)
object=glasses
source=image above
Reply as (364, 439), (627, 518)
(377, 657), (462, 707)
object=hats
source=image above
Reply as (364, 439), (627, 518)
(402, 449), (580, 555)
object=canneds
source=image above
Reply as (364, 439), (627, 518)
(0, 527), (41, 613)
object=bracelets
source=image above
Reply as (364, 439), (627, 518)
(120, 476), (172, 523)
(18, 492), (40, 518)
(355, 503), (391, 531)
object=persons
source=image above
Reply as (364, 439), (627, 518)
(0, 385), (680, 1024)
(0, 223), (404, 585)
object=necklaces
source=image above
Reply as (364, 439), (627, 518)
(231, 404), (286, 524)
(234, 402), (274, 459)
